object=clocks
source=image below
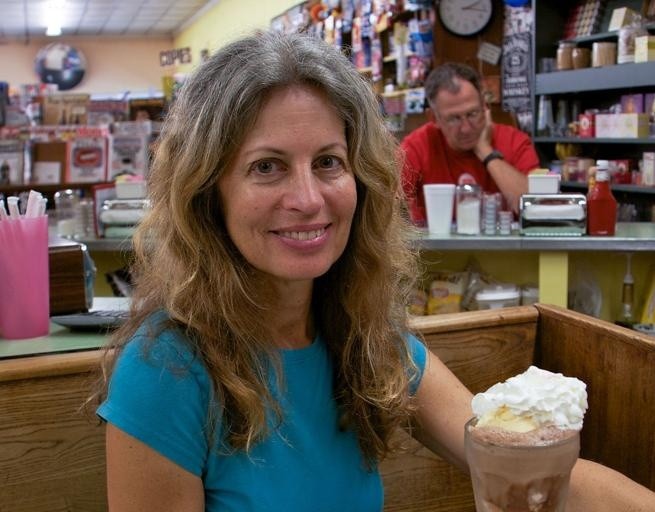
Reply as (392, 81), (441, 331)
(436, 1), (496, 39)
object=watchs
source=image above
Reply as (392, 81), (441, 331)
(483, 151), (504, 167)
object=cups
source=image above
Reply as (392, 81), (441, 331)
(423, 183), (456, 239)
(463, 416), (582, 512)
(0, 217), (49, 341)
(572, 42), (617, 68)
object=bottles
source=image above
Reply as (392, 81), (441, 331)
(586, 159), (617, 235)
(453, 184), (512, 237)
(615, 276), (638, 328)
(53, 190), (83, 243)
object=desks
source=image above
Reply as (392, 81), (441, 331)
(404, 223), (655, 309)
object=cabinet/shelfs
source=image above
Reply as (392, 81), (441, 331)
(530, 23), (655, 194)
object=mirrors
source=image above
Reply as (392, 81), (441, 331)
(33, 42), (86, 90)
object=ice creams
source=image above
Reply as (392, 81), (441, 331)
(463, 366), (589, 512)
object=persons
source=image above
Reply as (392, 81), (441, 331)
(95, 30), (655, 512)
(394, 61), (541, 230)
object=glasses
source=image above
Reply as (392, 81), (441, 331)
(434, 102), (483, 127)
